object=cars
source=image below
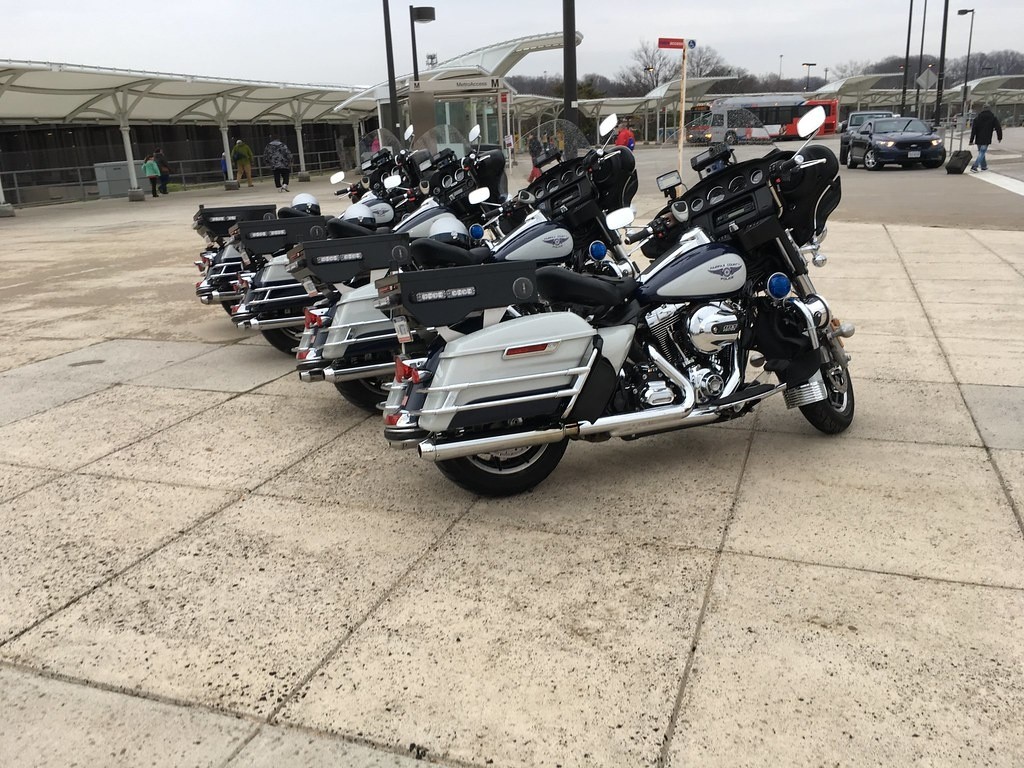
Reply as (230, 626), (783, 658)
(1001, 115), (1024, 128)
(847, 117), (946, 170)
(921, 112), (976, 129)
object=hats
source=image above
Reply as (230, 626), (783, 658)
(269, 134), (281, 142)
(984, 104), (991, 111)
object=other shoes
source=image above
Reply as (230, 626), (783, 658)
(970, 166), (979, 173)
(152, 195), (158, 197)
(277, 187), (282, 194)
(282, 184), (290, 193)
(158, 187), (163, 193)
(162, 191), (168, 194)
(981, 166), (988, 172)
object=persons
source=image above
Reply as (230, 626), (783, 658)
(528, 132), (542, 163)
(263, 136), (293, 192)
(154, 148), (172, 194)
(616, 124), (635, 152)
(231, 140), (253, 187)
(969, 103), (1002, 172)
(746, 126), (752, 144)
(372, 136), (380, 152)
(221, 153), (227, 181)
(542, 132), (563, 153)
(141, 155), (161, 197)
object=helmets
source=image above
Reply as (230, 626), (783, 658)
(343, 204), (378, 232)
(428, 216), (471, 252)
(291, 192), (321, 218)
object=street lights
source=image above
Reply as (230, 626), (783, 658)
(923, 64), (933, 122)
(643, 67), (654, 97)
(956, 8), (975, 117)
(408, 5), (436, 81)
(802, 63), (816, 92)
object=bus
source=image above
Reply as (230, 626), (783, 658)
(685, 96), (839, 147)
(658, 127), (678, 141)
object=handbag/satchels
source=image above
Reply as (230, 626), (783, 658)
(161, 156), (170, 173)
(627, 129), (634, 150)
(233, 145), (241, 162)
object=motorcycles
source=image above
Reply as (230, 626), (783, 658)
(292, 113), (640, 415)
(383, 107), (855, 497)
(191, 126), (509, 357)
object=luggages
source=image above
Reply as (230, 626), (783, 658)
(946, 143), (974, 175)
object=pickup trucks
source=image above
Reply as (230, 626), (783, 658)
(840, 111), (900, 166)
